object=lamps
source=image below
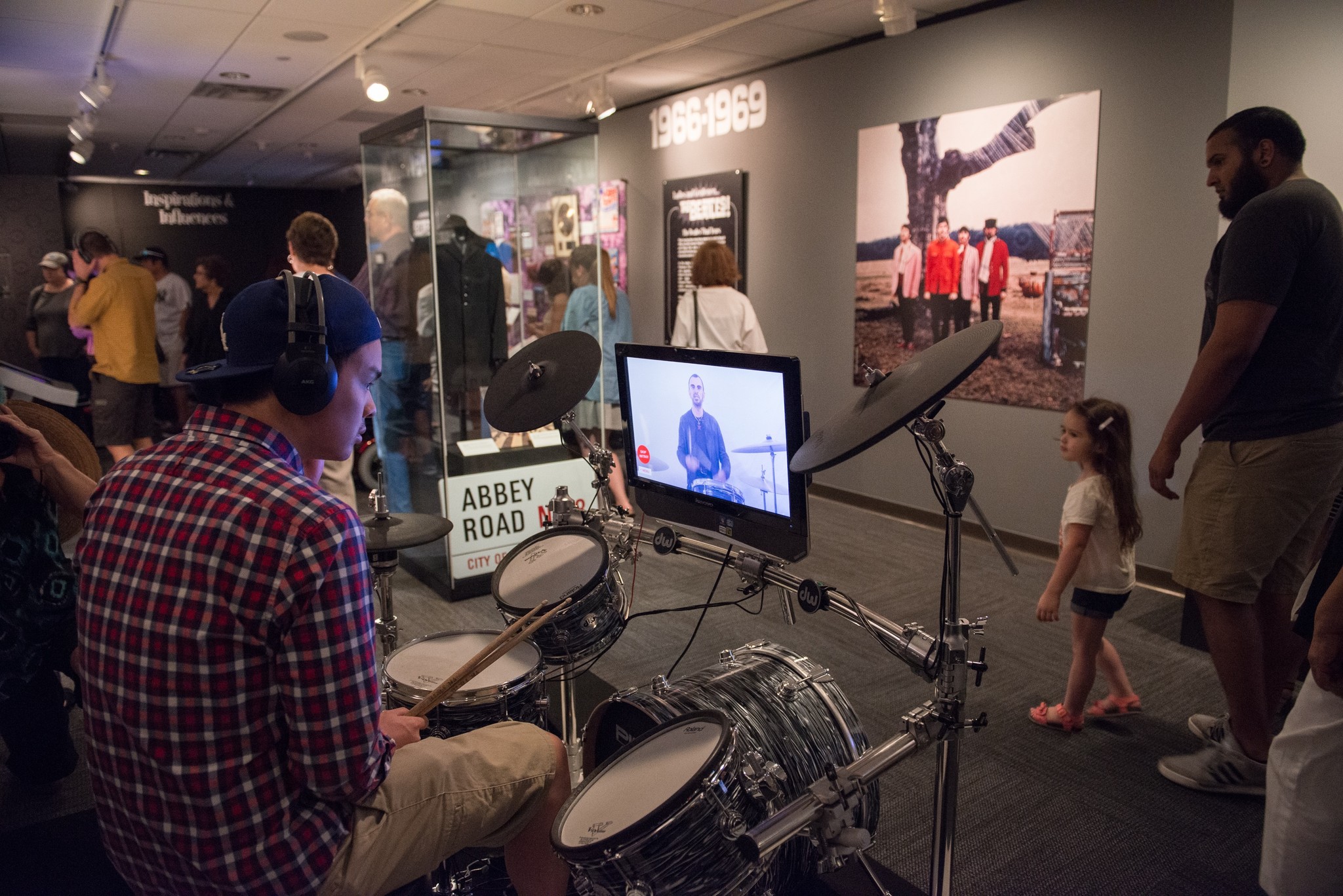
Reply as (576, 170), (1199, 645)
(584, 75), (616, 119)
(63, 56), (116, 164)
(877, 0), (920, 39)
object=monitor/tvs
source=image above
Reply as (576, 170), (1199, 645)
(612, 342), (811, 561)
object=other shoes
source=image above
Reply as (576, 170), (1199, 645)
(896, 340), (914, 350)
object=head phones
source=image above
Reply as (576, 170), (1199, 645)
(270, 268), (340, 417)
(72, 225), (119, 263)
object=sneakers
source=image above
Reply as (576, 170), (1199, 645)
(1186, 712), (1231, 744)
(1156, 737), (1267, 795)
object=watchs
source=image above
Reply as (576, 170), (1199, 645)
(73, 276), (88, 287)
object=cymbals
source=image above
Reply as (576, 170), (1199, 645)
(483, 329), (603, 434)
(786, 321), (1006, 480)
(357, 512), (454, 552)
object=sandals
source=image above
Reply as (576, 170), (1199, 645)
(1028, 702), (1086, 733)
(1085, 694), (1141, 722)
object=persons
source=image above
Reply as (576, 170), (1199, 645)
(1258, 507), (1343, 895)
(22, 251), (88, 428)
(72, 265), (573, 893)
(69, 232), (161, 465)
(174, 256), (234, 416)
(669, 239), (770, 354)
(676, 372), (731, 492)
(525, 255), (571, 343)
(1028, 396), (1144, 733)
(2, 402), (102, 786)
(128, 246), (194, 432)
(883, 216), (1011, 363)
(286, 211), (357, 520)
(351, 189), (419, 519)
(1147, 104), (1343, 796)
(559, 243), (637, 520)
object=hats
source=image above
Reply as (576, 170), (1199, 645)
(984, 219), (997, 229)
(133, 245), (167, 261)
(36, 251), (68, 270)
(175, 274), (385, 386)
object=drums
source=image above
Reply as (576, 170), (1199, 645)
(488, 529), (630, 670)
(572, 640), (882, 878)
(373, 630), (555, 740)
(548, 710), (790, 896)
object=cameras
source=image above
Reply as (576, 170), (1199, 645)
(0, 421), (21, 460)
(0, 359), (78, 407)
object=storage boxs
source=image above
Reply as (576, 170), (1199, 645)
(360, 101), (607, 604)
